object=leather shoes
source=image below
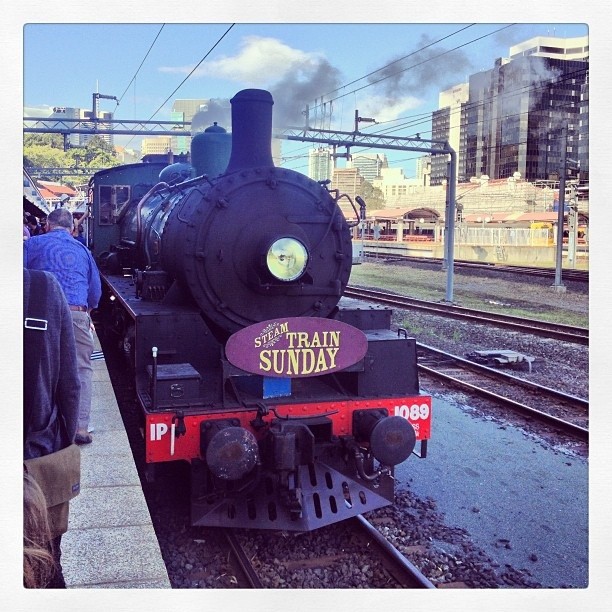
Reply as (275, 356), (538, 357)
(75, 430), (92, 445)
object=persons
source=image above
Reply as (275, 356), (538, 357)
(22, 225), (83, 588)
(23, 211), (48, 240)
(23, 209), (102, 444)
(74, 224), (87, 246)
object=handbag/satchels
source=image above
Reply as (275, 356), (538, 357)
(24, 269), (81, 539)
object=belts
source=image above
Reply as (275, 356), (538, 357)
(69, 305), (87, 312)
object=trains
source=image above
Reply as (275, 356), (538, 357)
(80, 89), (432, 534)
